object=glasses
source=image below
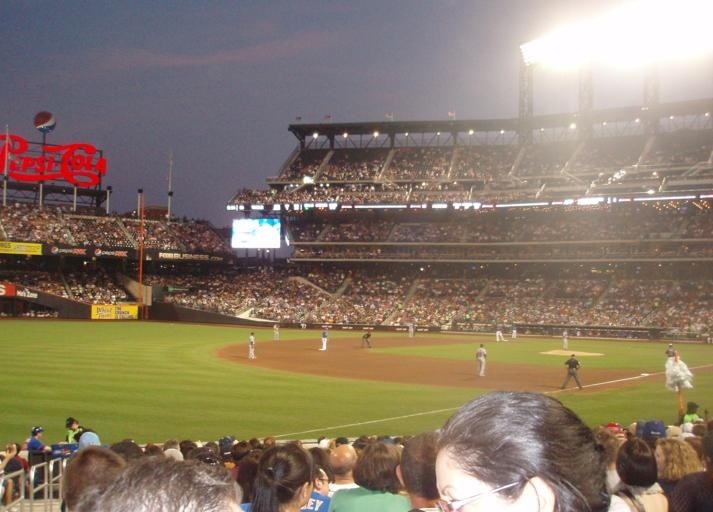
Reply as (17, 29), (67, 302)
(434, 478), (524, 512)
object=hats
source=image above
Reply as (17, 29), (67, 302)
(643, 420), (665, 438)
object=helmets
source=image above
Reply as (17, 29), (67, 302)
(31, 424), (44, 434)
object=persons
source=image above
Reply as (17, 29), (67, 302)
(559, 353), (583, 389)
(318, 328), (327, 351)
(226, 144), (712, 343)
(665, 344), (677, 362)
(0, 389), (712, 510)
(0, 196), (226, 320)
(248, 332), (256, 358)
(473, 343), (488, 377)
(359, 330), (372, 349)
